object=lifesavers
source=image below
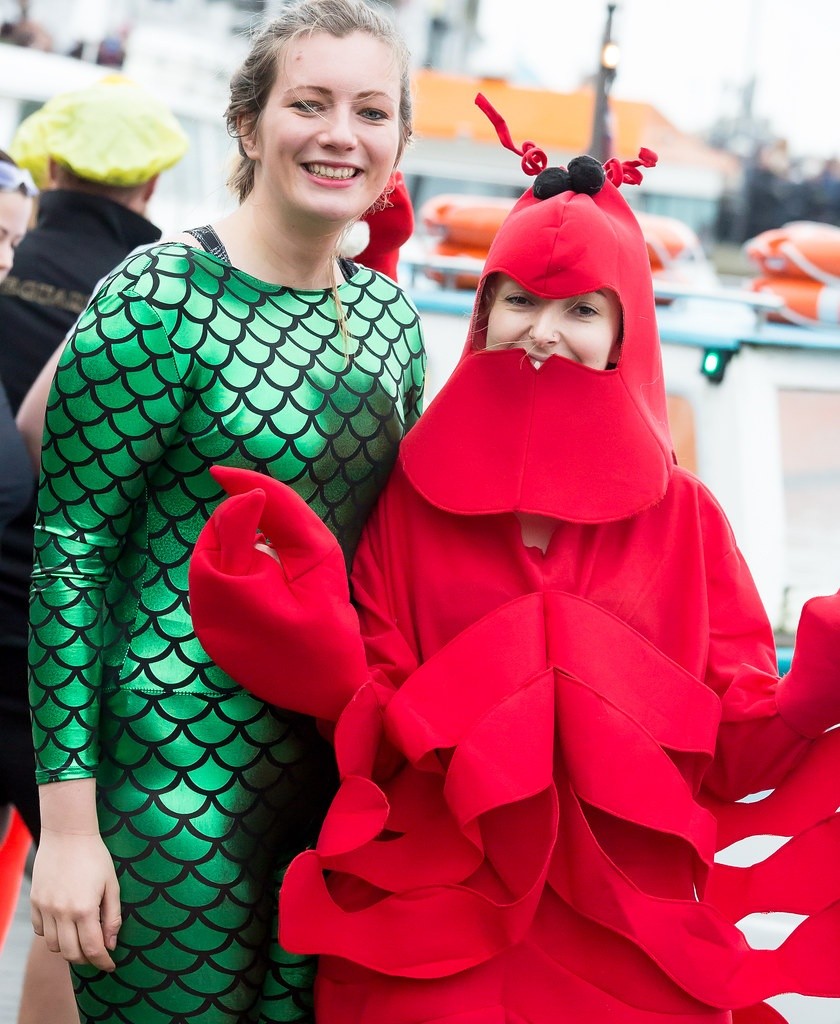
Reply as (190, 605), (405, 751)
(739, 213), (840, 327)
(420, 185), (699, 309)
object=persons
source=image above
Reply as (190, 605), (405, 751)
(0, 69), (197, 1024)
(188, 93), (840, 1024)
(25, 3), (428, 1024)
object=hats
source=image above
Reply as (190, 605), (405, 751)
(15, 74), (188, 197)
